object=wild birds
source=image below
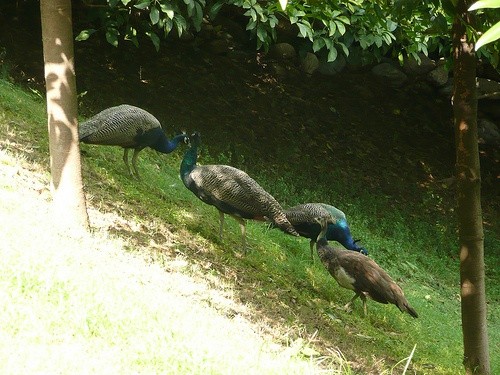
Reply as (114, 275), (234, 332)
(79, 104), (191, 174)
(314, 213), (419, 319)
(263, 202), (372, 261)
(179, 130), (300, 257)
